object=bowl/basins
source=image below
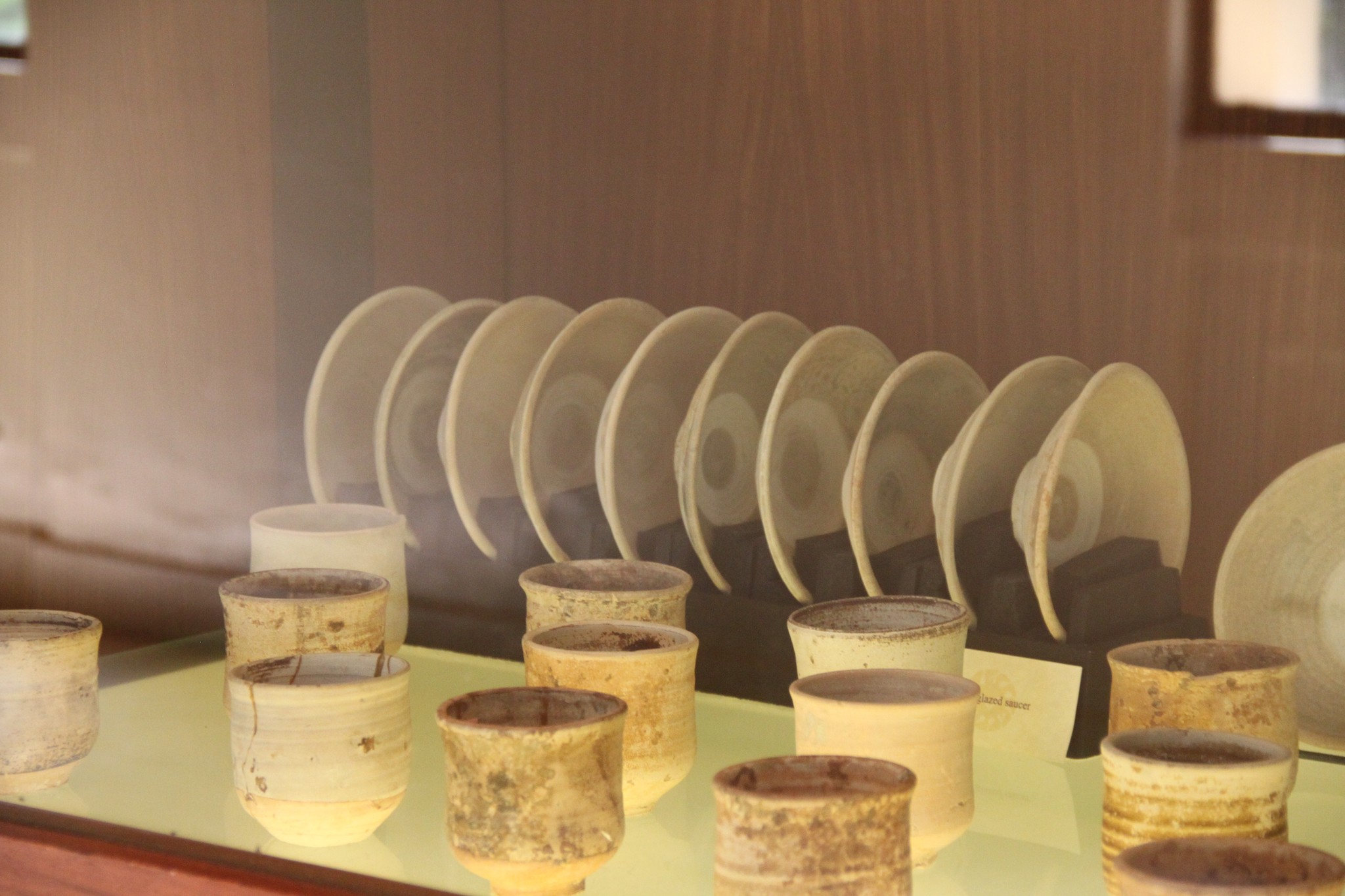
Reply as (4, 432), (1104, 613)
(373, 295), (503, 552)
(592, 305), (746, 561)
(1212, 444), (1345, 758)
(752, 323), (901, 606)
(436, 288), (581, 563)
(505, 298), (666, 563)
(671, 311), (813, 594)
(929, 356), (1093, 634)
(1008, 361), (1191, 644)
(303, 282), (453, 503)
(840, 349), (992, 604)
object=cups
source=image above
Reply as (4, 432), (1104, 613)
(520, 618), (699, 818)
(248, 503), (409, 660)
(224, 653), (411, 847)
(517, 558), (694, 631)
(709, 754), (919, 896)
(218, 568), (390, 655)
(786, 595), (971, 677)
(435, 687), (626, 896)
(1098, 732), (1298, 896)
(0, 610), (104, 794)
(1106, 636), (1302, 800)
(788, 670), (981, 866)
(1113, 836), (1345, 895)
